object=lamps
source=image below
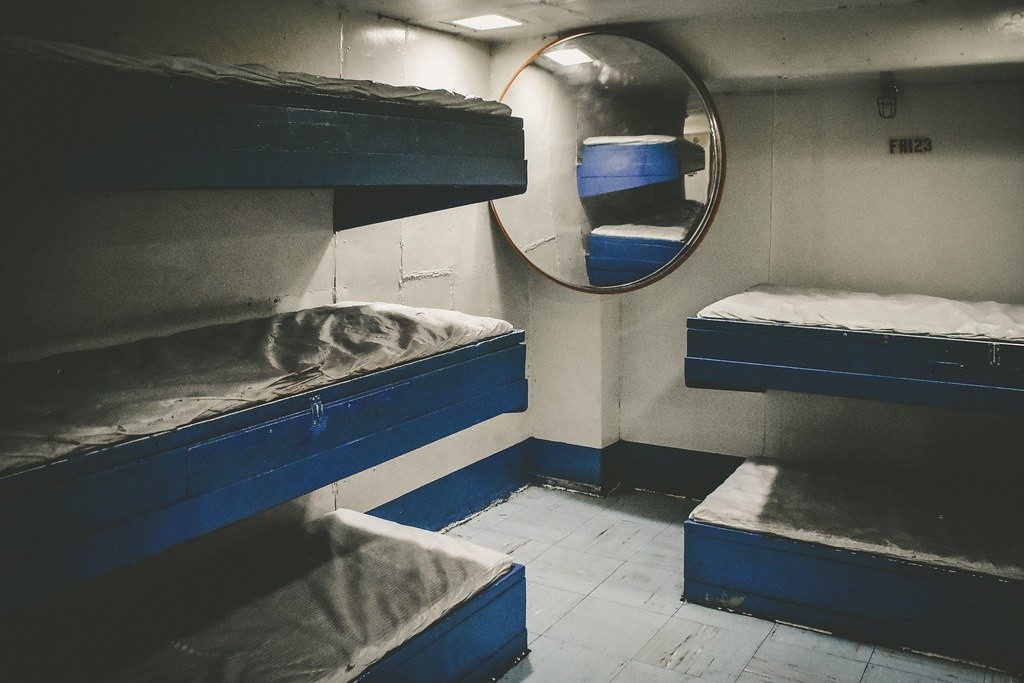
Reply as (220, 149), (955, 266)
(877, 96), (897, 119)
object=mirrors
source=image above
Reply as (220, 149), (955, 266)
(487, 29), (727, 297)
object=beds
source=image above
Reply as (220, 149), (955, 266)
(0, 297), (531, 621)
(578, 134), (705, 199)
(684, 281), (1024, 421)
(0, 507), (528, 683)
(0, 33), (528, 193)
(683, 459), (1024, 679)
(586, 199), (703, 287)
